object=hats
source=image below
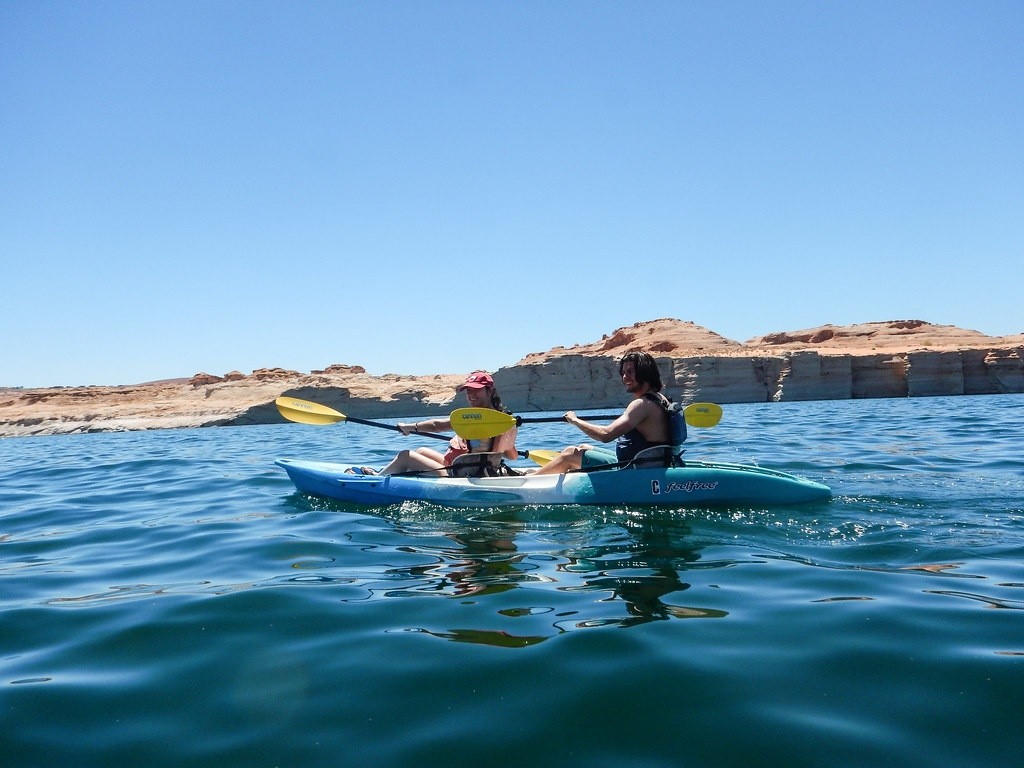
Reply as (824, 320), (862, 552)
(460, 372), (493, 392)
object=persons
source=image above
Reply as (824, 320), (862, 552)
(344, 370), (518, 478)
(514, 351), (687, 475)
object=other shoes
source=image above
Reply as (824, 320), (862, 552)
(501, 464), (525, 477)
(344, 468), (356, 474)
(361, 466), (375, 475)
(484, 467), (498, 477)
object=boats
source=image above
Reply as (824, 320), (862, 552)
(274, 449), (833, 510)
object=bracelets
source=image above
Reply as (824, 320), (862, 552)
(415, 423), (419, 434)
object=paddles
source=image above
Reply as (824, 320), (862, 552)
(275, 396), (563, 467)
(449, 402), (723, 440)
